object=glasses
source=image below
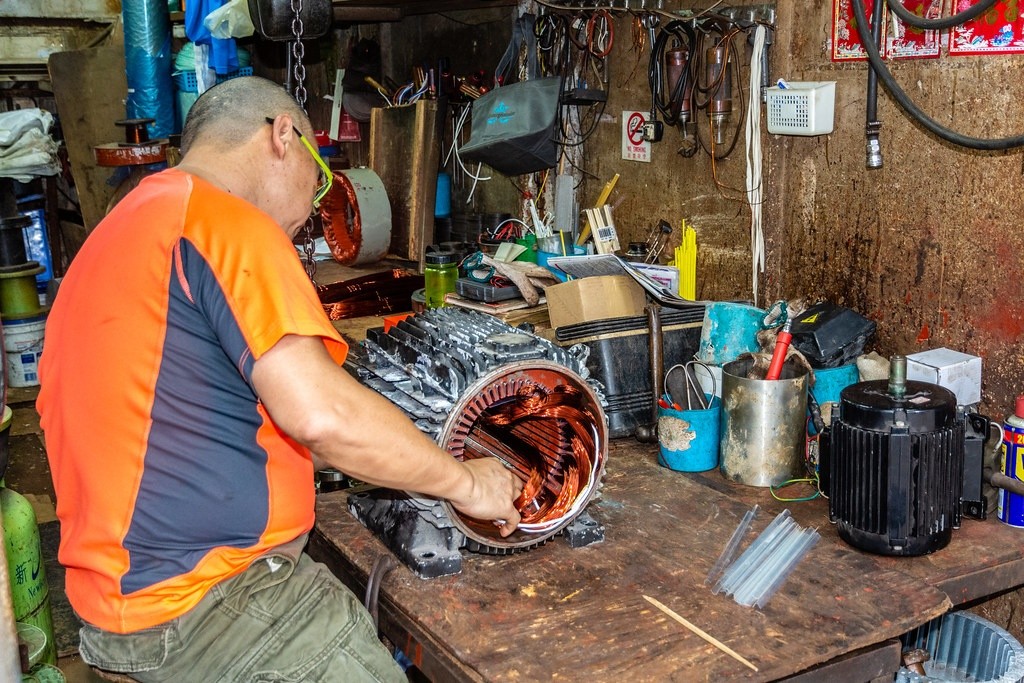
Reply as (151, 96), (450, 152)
(264, 116), (333, 203)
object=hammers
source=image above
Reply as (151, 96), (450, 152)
(634, 307), (664, 442)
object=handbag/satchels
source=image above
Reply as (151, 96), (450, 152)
(457, 12), (561, 176)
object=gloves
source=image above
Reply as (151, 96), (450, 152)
(465, 252), (562, 307)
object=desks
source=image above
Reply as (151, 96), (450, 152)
(303, 314), (1024, 683)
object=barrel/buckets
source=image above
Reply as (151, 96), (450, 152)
(1, 319), (46, 388)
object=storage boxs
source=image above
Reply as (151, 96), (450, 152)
(905, 348), (982, 408)
(555, 303), (746, 436)
(546, 274), (645, 329)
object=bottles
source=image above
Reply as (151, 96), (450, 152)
(423, 252), (461, 309)
(996, 394), (1023, 528)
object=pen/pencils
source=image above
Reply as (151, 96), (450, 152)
(659, 399), (669, 408)
(674, 403), (681, 410)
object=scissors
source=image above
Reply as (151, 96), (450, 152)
(664, 360), (717, 412)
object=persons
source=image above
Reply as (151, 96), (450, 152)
(35, 75), (524, 683)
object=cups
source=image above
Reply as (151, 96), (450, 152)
(657, 392), (722, 474)
(478, 231), (587, 283)
(693, 302), (859, 488)
(434, 172), (451, 218)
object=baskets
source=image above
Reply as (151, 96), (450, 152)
(173, 67), (255, 92)
